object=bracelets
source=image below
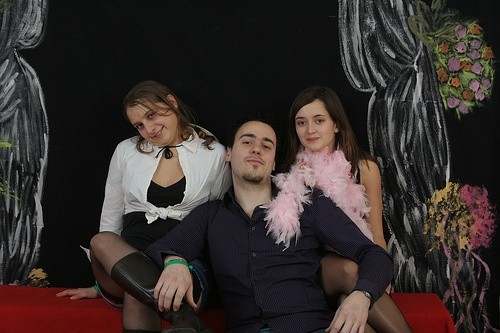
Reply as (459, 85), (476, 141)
(164, 258), (193, 271)
(362, 290), (372, 299)
(93, 286), (101, 293)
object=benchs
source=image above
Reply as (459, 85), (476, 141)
(0, 285), (455, 333)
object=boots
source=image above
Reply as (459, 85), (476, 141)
(112, 251), (213, 332)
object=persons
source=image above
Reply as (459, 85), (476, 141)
(135, 116), (396, 333)
(55, 79), (233, 333)
(285, 84), (412, 333)
